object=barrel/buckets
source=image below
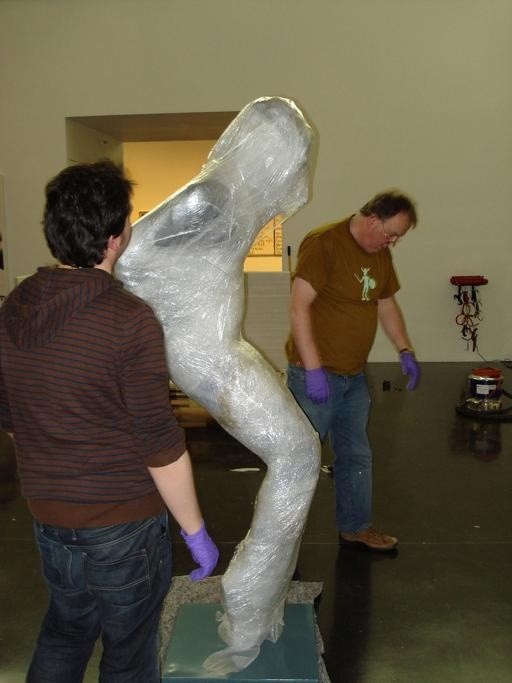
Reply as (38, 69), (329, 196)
(469, 416), (501, 462)
(463, 367), (504, 411)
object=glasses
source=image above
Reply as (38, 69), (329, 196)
(382, 221), (397, 242)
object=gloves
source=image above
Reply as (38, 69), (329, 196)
(399, 352), (419, 392)
(179, 521), (219, 582)
(305, 367), (328, 407)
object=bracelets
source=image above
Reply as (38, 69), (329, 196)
(400, 347), (415, 353)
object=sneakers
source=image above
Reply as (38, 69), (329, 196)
(341, 524), (397, 550)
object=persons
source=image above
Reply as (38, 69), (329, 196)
(285, 190), (422, 550)
(0, 160), (219, 683)
